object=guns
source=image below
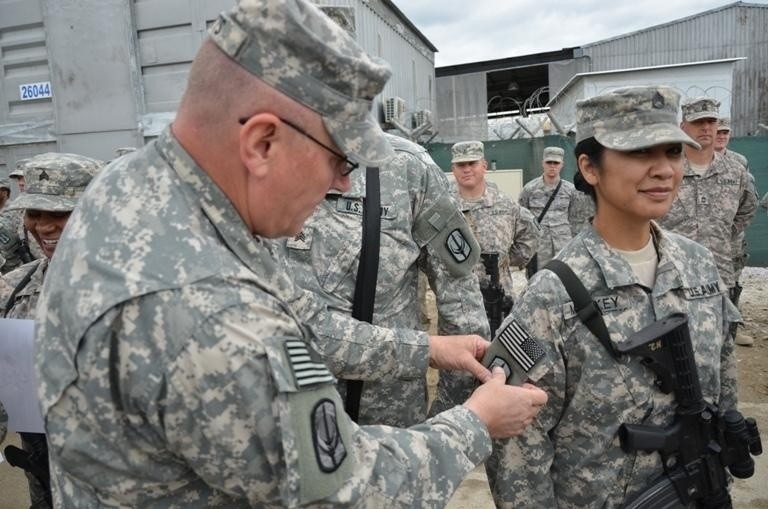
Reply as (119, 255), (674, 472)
(617, 312), (763, 509)
(478, 250), (514, 343)
(3, 431), (53, 509)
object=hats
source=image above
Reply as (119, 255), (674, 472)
(8, 159), (32, 177)
(543, 147), (564, 163)
(3, 153), (105, 214)
(209, 0), (396, 168)
(451, 142), (484, 163)
(0, 178), (10, 191)
(107, 148), (138, 165)
(576, 86), (701, 152)
(716, 118), (731, 131)
(682, 96), (721, 121)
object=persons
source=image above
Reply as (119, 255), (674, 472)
(657, 96), (760, 421)
(0, 151), (106, 509)
(427, 139), (545, 421)
(0, 177), (32, 278)
(11, 158), (32, 193)
(517, 145), (577, 285)
(474, 84), (744, 509)
(263, 7), (493, 429)
(35, 0), (547, 509)
(713, 117), (754, 341)
